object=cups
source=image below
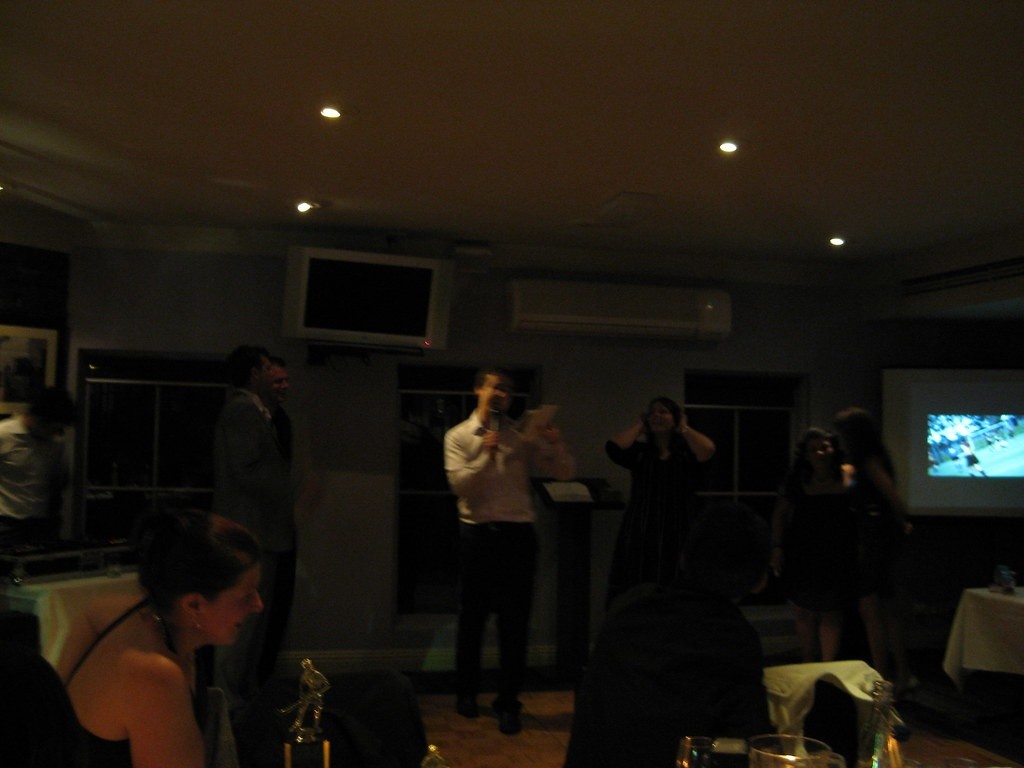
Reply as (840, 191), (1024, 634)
(283, 740), (330, 768)
(675, 736), (749, 768)
(1001, 570), (1017, 594)
(995, 565), (1008, 583)
(746, 734), (846, 768)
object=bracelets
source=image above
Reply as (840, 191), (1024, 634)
(769, 542), (783, 548)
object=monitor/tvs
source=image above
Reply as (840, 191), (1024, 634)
(280, 243), (454, 350)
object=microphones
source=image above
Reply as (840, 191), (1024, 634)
(489, 407), (504, 462)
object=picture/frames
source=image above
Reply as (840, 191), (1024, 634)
(0, 324), (59, 420)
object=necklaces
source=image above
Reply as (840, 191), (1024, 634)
(153, 608), (179, 655)
(813, 477), (833, 485)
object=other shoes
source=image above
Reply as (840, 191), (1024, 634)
(458, 696), (479, 719)
(500, 709), (522, 734)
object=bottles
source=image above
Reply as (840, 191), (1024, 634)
(420, 744), (451, 768)
(856, 680), (905, 768)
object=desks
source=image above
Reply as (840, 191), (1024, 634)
(940, 585), (1024, 696)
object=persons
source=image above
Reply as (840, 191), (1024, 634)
(864, 680), (909, 768)
(0, 389), (85, 577)
(604, 394), (719, 609)
(440, 363), (575, 734)
(564, 500), (772, 768)
(288, 657), (331, 733)
(216, 348), (297, 699)
(419, 742), (452, 768)
(19, 506), (266, 768)
(767, 408), (920, 702)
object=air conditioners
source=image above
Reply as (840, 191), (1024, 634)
(506, 276), (732, 348)
